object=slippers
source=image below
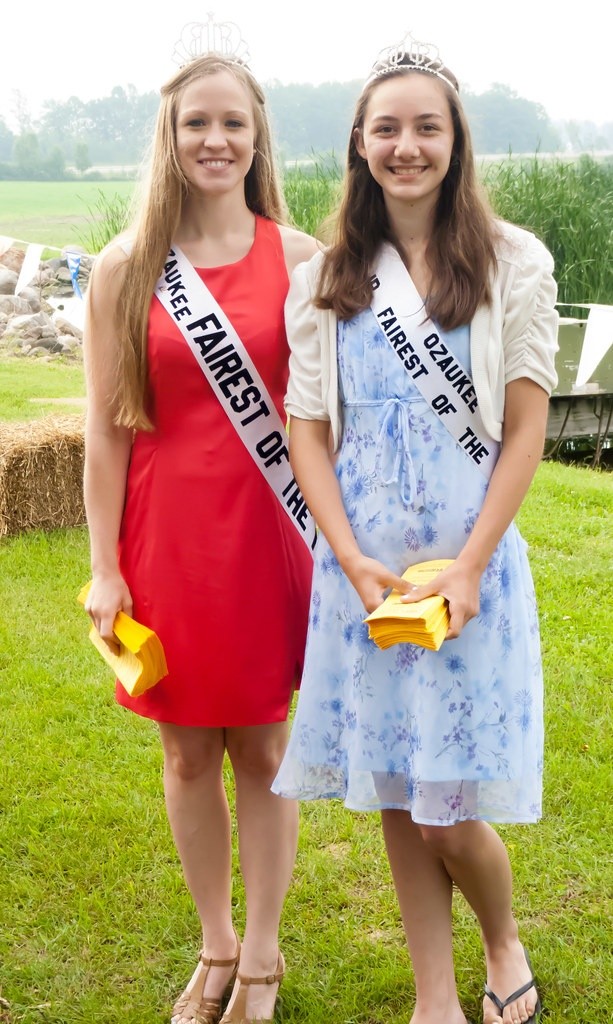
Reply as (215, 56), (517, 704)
(482, 977), (541, 1024)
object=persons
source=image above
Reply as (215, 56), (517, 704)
(283, 50), (561, 1024)
(80, 53), (340, 1024)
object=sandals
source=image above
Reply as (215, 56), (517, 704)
(221, 948), (285, 1024)
(170, 927), (241, 1024)
